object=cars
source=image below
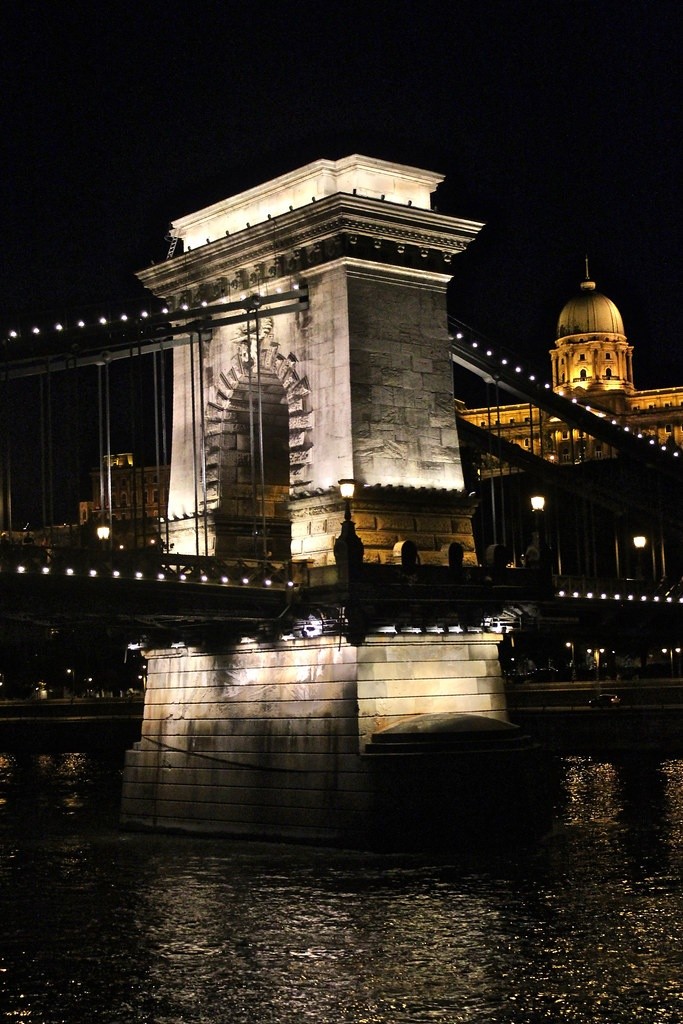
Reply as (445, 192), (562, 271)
(587, 693), (623, 708)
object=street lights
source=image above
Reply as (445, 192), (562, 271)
(333, 478), (365, 583)
(529, 493), (548, 602)
(566, 641), (578, 681)
(67, 669), (75, 698)
(587, 649), (606, 668)
(633, 534), (648, 601)
(661, 647), (682, 679)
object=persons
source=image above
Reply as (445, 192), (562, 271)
(23, 533), (33, 544)
(258, 550), (273, 568)
(0, 533), (9, 543)
